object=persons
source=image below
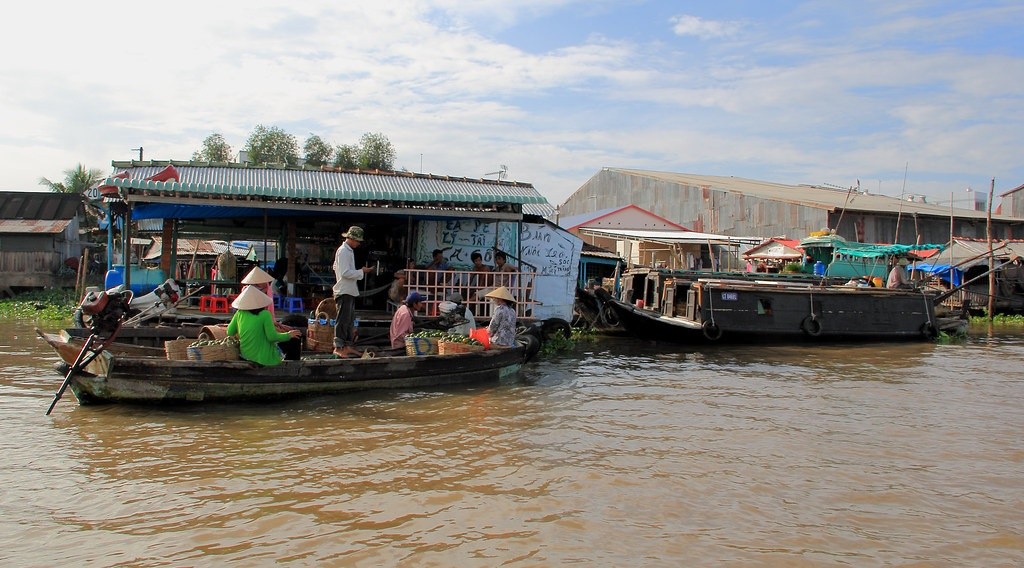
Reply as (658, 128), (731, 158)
(391, 249), (521, 350)
(225, 266), (302, 365)
(332, 225), (376, 358)
(885, 257), (917, 291)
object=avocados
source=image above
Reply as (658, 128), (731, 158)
(189, 336), (241, 347)
(405, 331), (485, 346)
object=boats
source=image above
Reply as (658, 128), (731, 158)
(575, 267), (968, 340)
(32, 288), (541, 415)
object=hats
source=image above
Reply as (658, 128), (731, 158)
(230, 285), (273, 310)
(485, 286), (518, 304)
(897, 258), (911, 265)
(402, 292), (426, 303)
(241, 265), (274, 284)
(446, 293), (463, 303)
(341, 226), (365, 241)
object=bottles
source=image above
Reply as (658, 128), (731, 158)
(813, 261), (824, 276)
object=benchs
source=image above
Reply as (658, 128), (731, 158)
(410, 289), (446, 316)
(387, 300), (401, 315)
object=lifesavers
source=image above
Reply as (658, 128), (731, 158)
(922, 322), (939, 341)
(600, 304), (622, 328)
(702, 319), (722, 341)
(594, 288), (613, 307)
(518, 334), (540, 363)
(541, 317), (571, 342)
(803, 316), (823, 337)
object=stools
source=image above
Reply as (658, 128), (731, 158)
(283, 297), (304, 314)
(200, 295), (215, 312)
(210, 297), (229, 313)
(227, 294), (239, 309)
(273, 295), (281, 308)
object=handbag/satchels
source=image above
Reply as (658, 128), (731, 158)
(469, 327), (489, 351)
